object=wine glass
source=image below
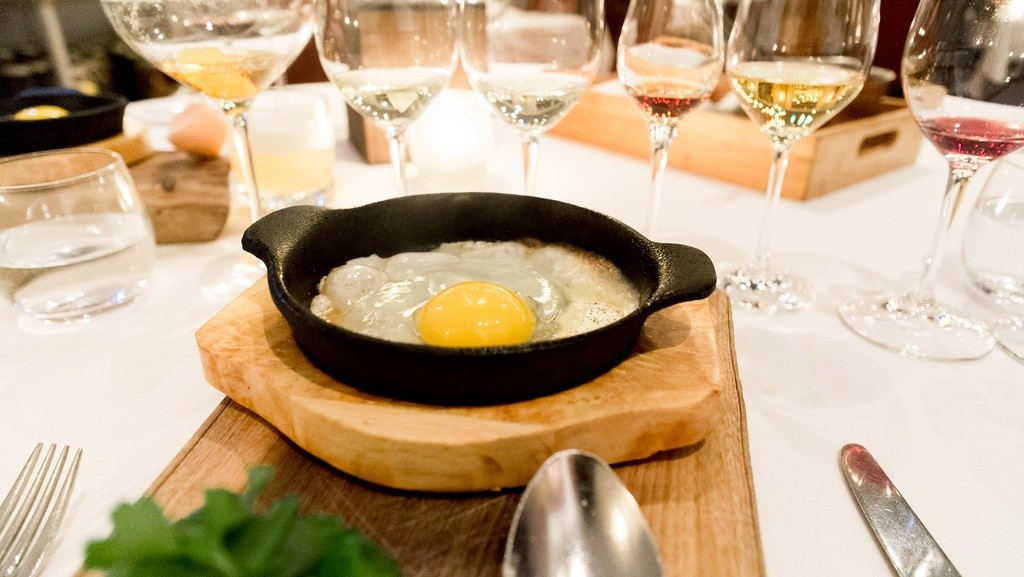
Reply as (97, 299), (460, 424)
(840, 0), (1023, 357)
(717, 0), (882, 312)
(97, 1), (317, 227)
(615, 1), (726, 245)
(313, 1), (463, 196)
(460, 0), (607, 196)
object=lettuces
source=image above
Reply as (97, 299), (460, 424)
(84, 466), (402, 577)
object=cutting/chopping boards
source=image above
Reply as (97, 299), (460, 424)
(0, 152), (230, 244)
(71, 289), (765, 577)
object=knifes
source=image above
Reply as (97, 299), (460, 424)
(835, 443), (961, 576)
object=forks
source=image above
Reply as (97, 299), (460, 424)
(0, 443), (83, 577)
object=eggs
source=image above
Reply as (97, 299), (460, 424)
(312, 240), (637, 347)
(13, 105), (68, 119)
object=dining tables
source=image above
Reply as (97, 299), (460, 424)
(0, 82), (1024, 577)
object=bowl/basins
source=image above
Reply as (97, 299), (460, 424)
(820, 64), (898, 131)
(0, 85), (127, 160)
(241, 192), (718, 408)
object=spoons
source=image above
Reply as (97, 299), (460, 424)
(501, 448), (662, 577)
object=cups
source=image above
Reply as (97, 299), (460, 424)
(961, 150), (1023, 304)
(0, 152), (157, 321)
